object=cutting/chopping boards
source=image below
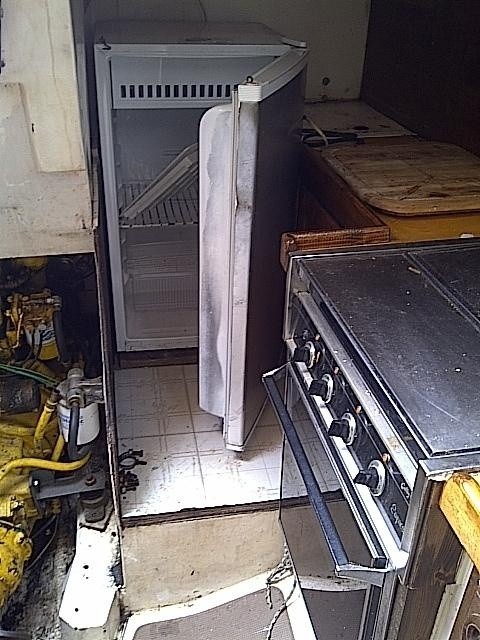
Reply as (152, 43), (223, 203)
(321, 139), (480, 217)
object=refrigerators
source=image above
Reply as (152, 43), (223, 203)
(93, 19), (308, 458)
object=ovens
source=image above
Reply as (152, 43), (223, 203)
(261, 234), (480, 640)
